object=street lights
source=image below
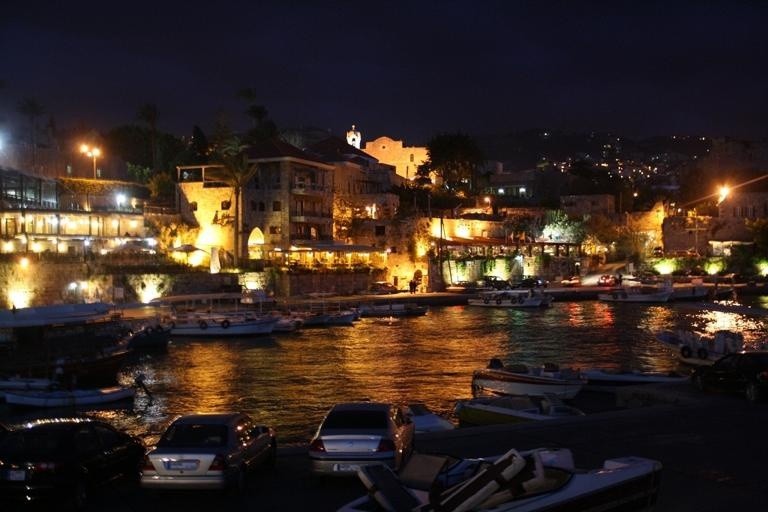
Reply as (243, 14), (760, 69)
(79, 143), (101, 179)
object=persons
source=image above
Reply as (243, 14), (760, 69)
(412, 281), (418, 294)
(409, 278), (415, 293)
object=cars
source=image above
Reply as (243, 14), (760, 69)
(0, 420), (148, 511)
(304, 400), (401, 478)
(139, 413), (278, 493)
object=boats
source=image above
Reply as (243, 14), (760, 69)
(0, 373), (155, 408)
(0, 302), (166, 390)
(598, 284), (674, 301)
(468, 280), (552, 309)
(273, 294), (430, 333)
(157, 290), (281, 336)
(341, 347), (768, 512)
